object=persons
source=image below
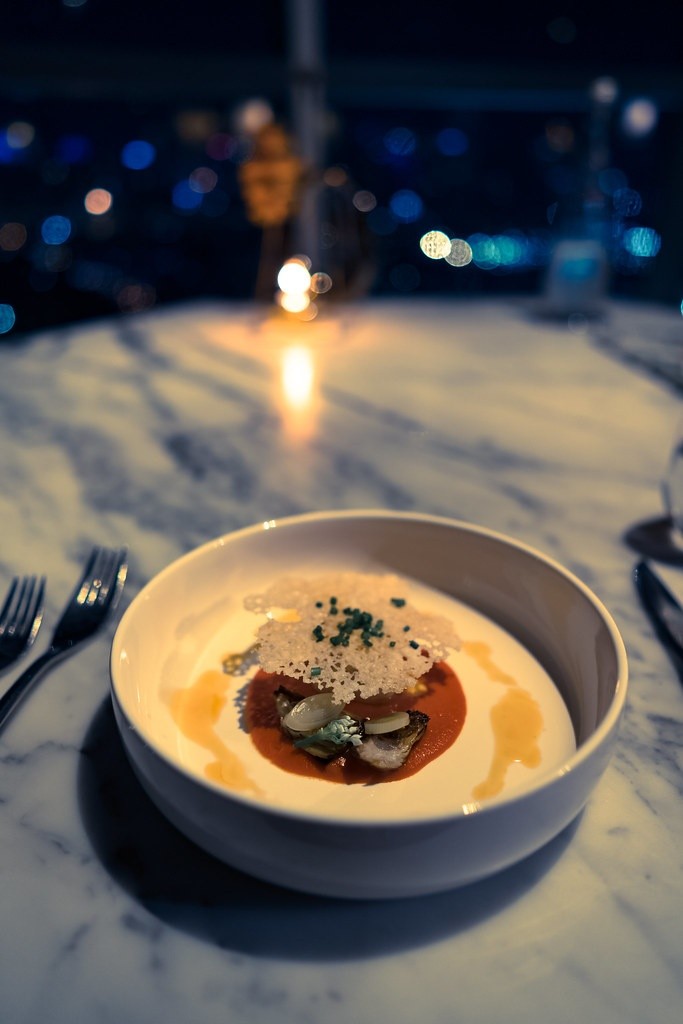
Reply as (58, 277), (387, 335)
(239, 125), (348, 300)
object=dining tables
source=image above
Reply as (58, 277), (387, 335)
(0, 299), (683, 1024)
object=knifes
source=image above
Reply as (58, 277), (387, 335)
(637, 560), (683, 686)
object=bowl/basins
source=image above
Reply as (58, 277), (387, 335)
(108, 507), (629, 899)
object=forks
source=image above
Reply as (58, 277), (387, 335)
(0, 575), (46, 672)
(0, 546), (128, 730)
